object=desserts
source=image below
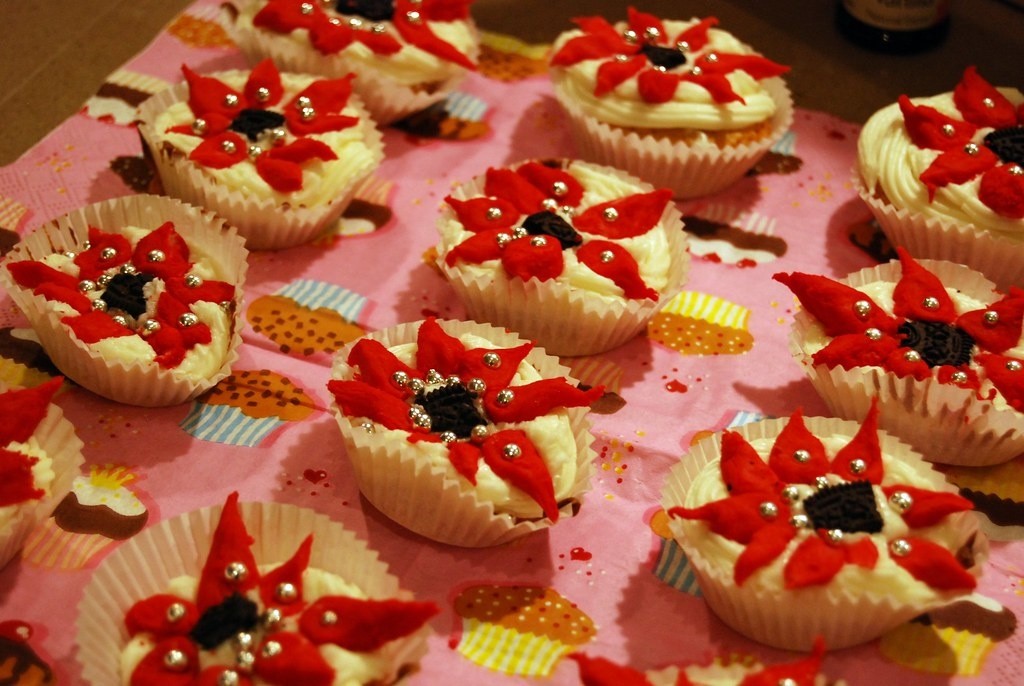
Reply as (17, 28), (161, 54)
(0, 0), (1024, 686)
